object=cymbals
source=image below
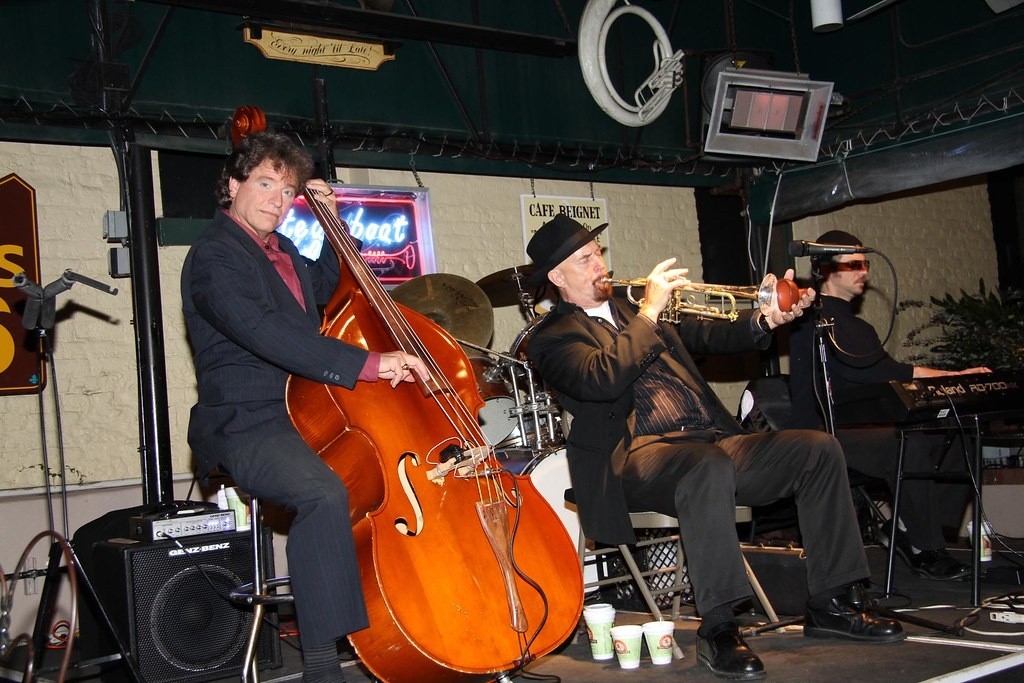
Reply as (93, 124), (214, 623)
(389, 272), (495, 354)
(476, 264), (553, 304)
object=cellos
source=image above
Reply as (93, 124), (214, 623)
(223, 105), (585, 683)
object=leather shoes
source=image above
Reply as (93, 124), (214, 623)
(803, 593), (907, 643)
(696, 622), (768, 681)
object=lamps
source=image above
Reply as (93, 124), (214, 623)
(810, 0), (843, 33)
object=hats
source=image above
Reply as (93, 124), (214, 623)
(527, 214), (609, 280)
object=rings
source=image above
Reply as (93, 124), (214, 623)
(325, 188), (333, 196)
(402, 364), (409, 371)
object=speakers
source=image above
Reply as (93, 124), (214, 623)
(75, 526), (283, 683)
(959, 467), (1024, 539)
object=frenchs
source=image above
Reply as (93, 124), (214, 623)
(577, 0), (686, 127)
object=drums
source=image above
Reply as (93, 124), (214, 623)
(467, 355), (519, 447)
(505, 312), (562, 401)
(487, 445), (610, 594)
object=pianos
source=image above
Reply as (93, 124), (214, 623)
(878, 370), (1024, 605)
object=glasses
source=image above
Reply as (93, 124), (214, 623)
(846, 260), (870, 271)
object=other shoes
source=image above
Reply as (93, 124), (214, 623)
(911, 548), (972, 581)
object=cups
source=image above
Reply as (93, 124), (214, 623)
(642, 621), (675, 664)
(611, 625), (643, 669)
(225, 486), (250, 531)
(967, 521), (991, 561)
(583, 604), (615, 660)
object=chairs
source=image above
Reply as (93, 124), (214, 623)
(560, 410), (786, 660)
(736, 373), (918, 577)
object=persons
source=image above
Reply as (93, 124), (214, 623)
(522, 213), (905, 683)
(180, 130), (560, 683)
(787, 230), (989, 582)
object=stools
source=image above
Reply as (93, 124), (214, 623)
(217, 466), (294, 683)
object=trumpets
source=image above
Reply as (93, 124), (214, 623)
(600, 273), (780, 324)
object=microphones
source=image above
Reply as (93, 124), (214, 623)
(788, 239), (875, 257)
